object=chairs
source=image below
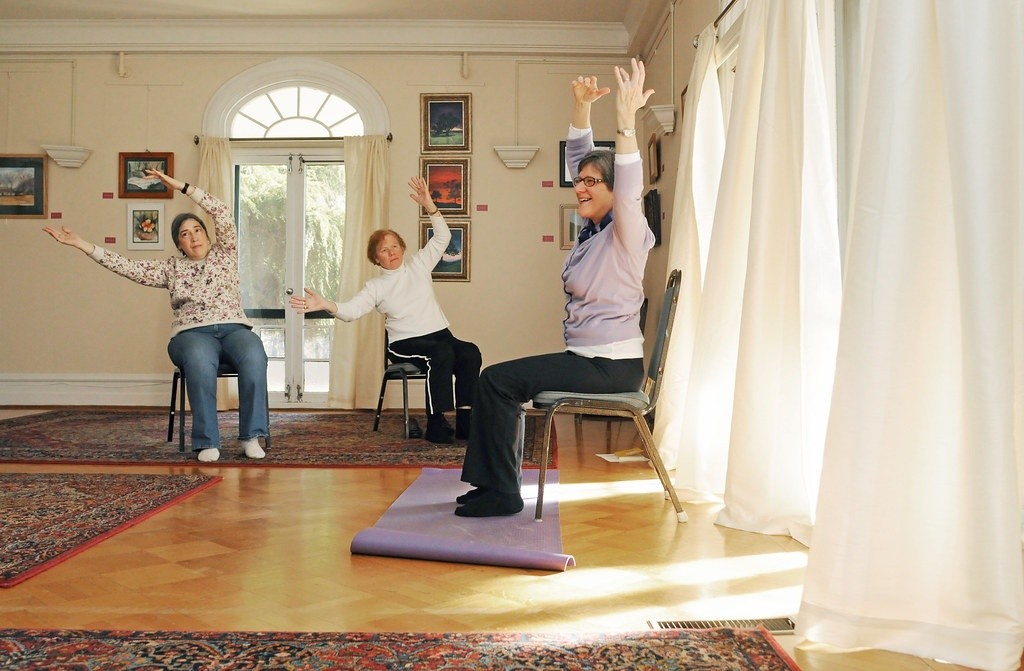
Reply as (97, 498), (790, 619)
(532, 268), (689, 523)
(373, 312), (429, 440)
(167, 367), (271, 452)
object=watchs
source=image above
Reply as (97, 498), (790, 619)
(616, 128), (638, 138)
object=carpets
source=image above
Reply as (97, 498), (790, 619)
(0, 473), (225, 589)
(0, 623), (804, 671)
(0, 409), (558, 469)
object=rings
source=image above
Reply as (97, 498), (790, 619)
(303, 306), (306, 309)
(622, 78), (629, 80)
(303, 301), (306, 305)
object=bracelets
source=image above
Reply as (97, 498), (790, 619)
(180, 181), (190, 194)
(427, 208), (439, 216)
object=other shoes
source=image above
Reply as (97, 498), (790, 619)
(440, 413), (454, 436)
(409, 418), (423, 438)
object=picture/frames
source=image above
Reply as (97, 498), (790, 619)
(420, 93), (472, 154)
(648, 133), (658, 185)
(644, 188), (661, 247)
(559, 141), (615, 187)
(127, 202), (165, 250)
(418, 157), (471, 218)
(419, 220), (471, 282)
(0, 153), (48, 219)
(118, 152), (174, 199)
(559, 204), (583, 250)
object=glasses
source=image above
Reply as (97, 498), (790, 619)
(573, 175), (606, 188)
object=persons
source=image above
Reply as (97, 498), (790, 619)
(288, 175), (482, 444)
(452, 56), (656, 519)
(39, 166), (268, 463)
